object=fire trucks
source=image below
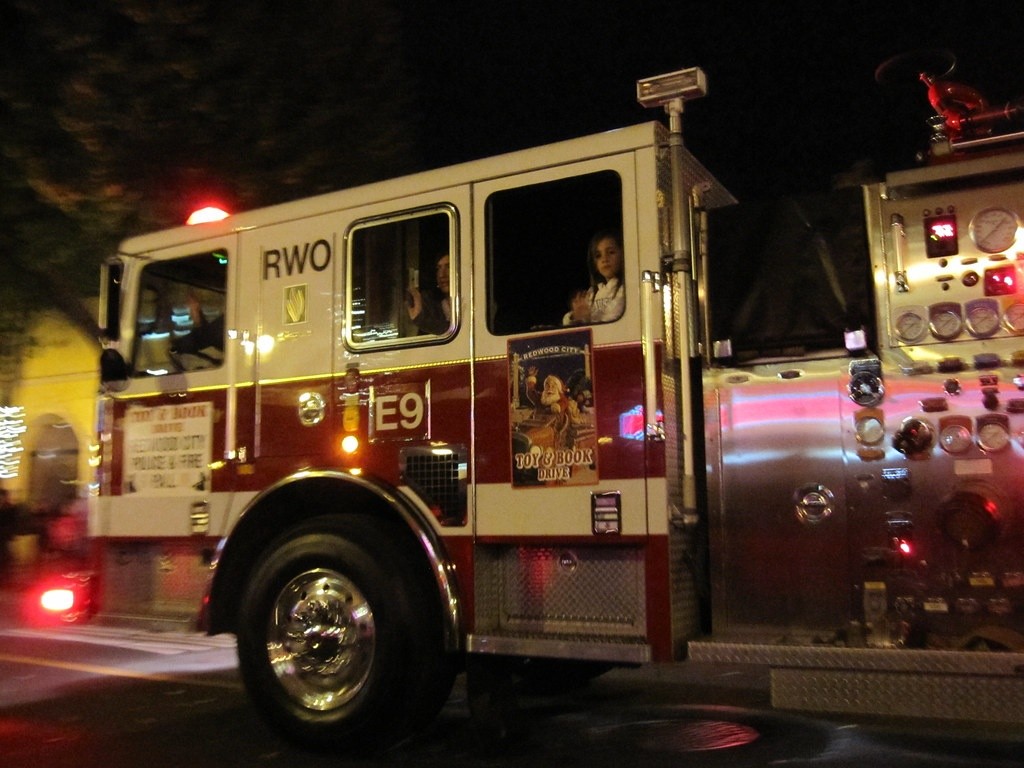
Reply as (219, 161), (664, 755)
(45, 64), (1020, 754)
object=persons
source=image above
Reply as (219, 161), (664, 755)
(525, 366), (579, 482)
(561, 227), (625, 327)
(402, 251), (452, 334)
(166, 283), (223, 357)
